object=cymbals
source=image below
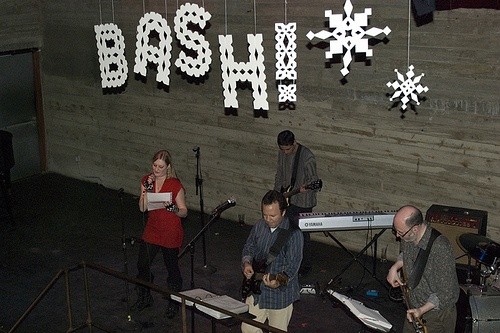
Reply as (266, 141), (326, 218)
(455, 232), (500, 267)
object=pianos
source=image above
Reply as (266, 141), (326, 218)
(297, 210), (401, 296)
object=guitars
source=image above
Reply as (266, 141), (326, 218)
(398, 264), (429, 333)
(241, 255), (290, 299)
(279, 178), (323, 207)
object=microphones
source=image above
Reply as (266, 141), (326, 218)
(211, 197), (235, 215)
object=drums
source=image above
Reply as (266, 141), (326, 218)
(455, 283), (500, 333)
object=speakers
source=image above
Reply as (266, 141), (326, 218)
(425, 205), (488, 268)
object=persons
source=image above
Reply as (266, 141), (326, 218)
(274, 130), (318, 277)
(130, 149), (187, 320)
(241, 190), (304, 333)
(387, 205), (460, 333)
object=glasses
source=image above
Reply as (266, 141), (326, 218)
(392, 224), (420, 238)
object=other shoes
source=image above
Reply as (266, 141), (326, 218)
(129, 295), (153, 313)
(164, 306), (178, 317)
